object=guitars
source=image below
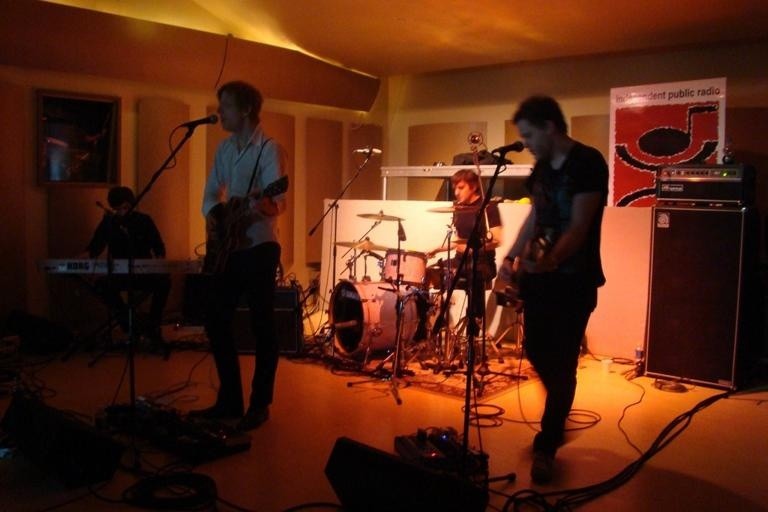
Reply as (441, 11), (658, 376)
(493, 238), (554, 307)
(206, 176), (289, 259)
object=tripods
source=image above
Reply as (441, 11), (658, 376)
(407, 300), (506, 378)
(493, 313), (536, 354)
(347, 298), (417, 404)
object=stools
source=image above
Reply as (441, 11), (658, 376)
(448, 281), (493, 367)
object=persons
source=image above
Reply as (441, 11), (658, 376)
(189, 81), (288, 431)
(426, 169), (504, 290)
(499, 93), (609, 486)
(88, 186), (173, 334)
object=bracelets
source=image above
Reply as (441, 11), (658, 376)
(505, 256), (515, 263)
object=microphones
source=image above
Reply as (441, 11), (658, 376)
(335, 319), (358, 330)
(180, 113), (218, 131)
(353, 146), (383, 156)
(493, 140), (525, 157)
(95, 196), (122, 219)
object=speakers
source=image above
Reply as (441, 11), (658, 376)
(641, 205), (759, 395)
(231, 287), (301, 356)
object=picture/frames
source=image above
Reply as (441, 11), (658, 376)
(33, 85), (122, 192)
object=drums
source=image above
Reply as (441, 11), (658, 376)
(380, 249), (427, 285)
(328, 279), (422, 370)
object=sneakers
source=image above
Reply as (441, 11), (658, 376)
(529, 428), (566, 487)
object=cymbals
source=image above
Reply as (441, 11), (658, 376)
(335, 241), (384, 253)
(359, 213), (400, 224)
(433, 205), (479, 216)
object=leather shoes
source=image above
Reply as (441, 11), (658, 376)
(236, 403), (271, 433)
(188, 398), (245, 422)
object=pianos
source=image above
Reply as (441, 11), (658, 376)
(40, 254), (204, 275)
